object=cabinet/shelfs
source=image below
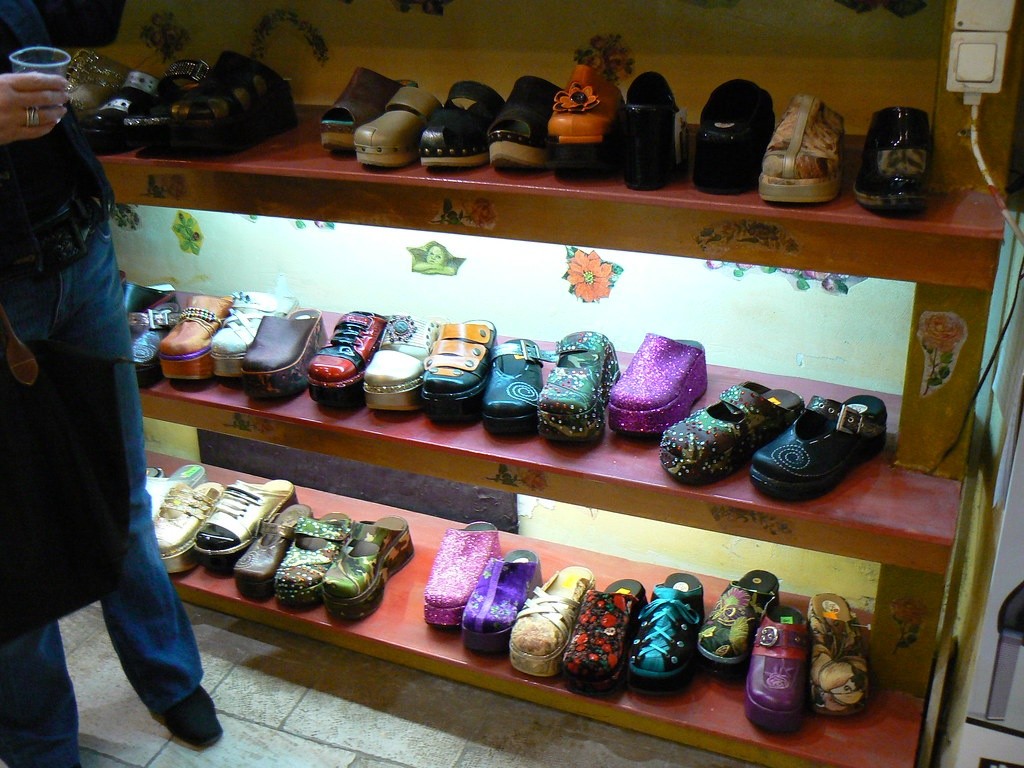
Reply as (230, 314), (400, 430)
(96, 104), (1007, 768)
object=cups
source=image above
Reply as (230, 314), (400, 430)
(9, 45), (71, 111)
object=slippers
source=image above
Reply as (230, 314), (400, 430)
(62, 48), (297, 146)
(853, 107), (930, 211)
(317, 48), (774, 195)
(123, 282), (887, 502)
(758, 92), (845, 203)
(145, 464), (870, 732)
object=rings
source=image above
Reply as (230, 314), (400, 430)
(26, 108), (39, 127)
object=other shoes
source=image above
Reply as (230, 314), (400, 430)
(162, 685), (223, 747)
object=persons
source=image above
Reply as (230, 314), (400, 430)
(0, 0), (222, 768)
